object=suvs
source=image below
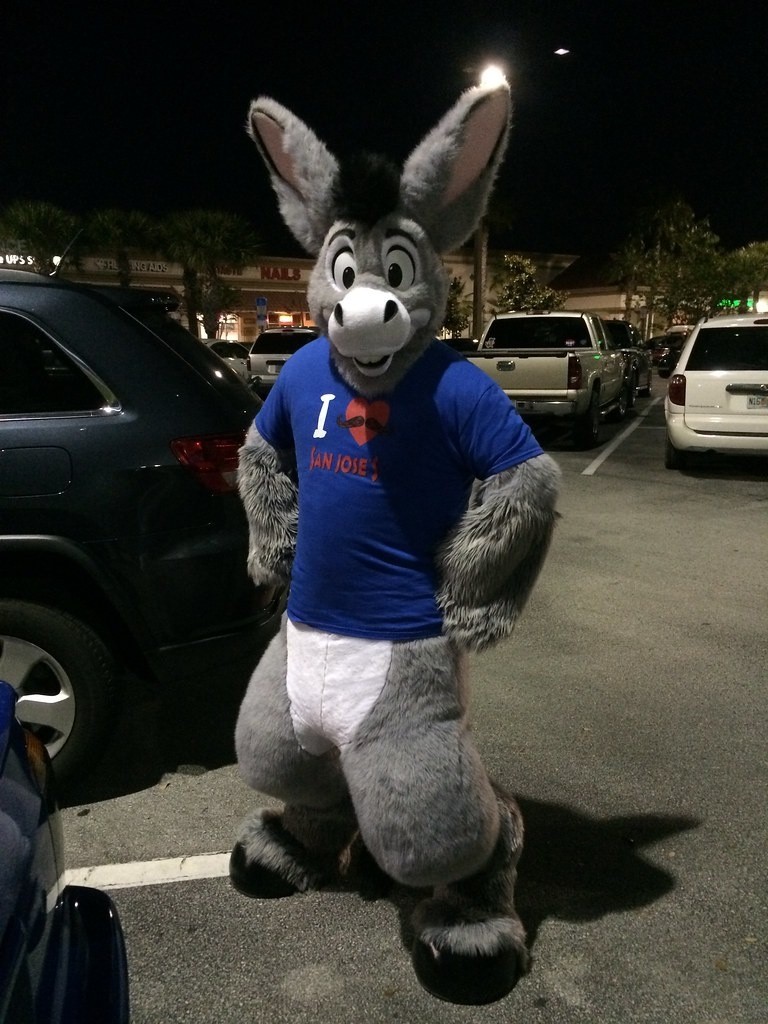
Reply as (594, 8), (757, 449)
(0, 264), (290, 811)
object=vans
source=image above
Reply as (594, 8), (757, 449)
(245, 327), (324, 398)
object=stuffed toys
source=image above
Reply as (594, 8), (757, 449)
(228, 76), (567, 1007)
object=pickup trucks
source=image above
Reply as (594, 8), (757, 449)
(457, 308), (638, 448)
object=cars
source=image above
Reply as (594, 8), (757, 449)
(638, 324), (695, 379)
(603, 318), (656, 408)
(197, 338), (251, 385)
(665, 312), (768, 471)
